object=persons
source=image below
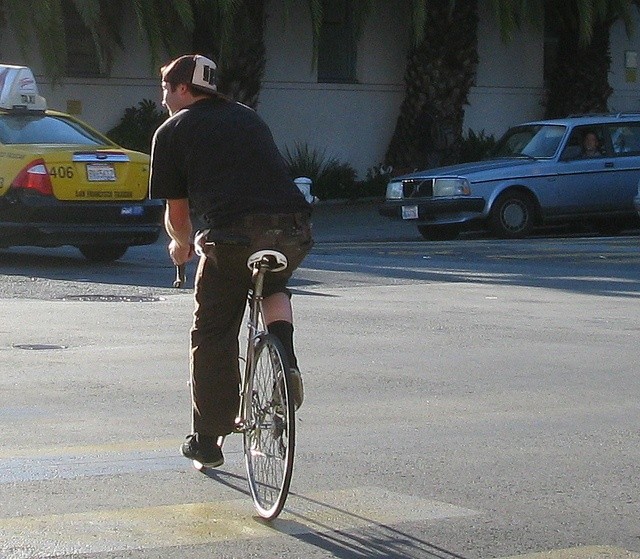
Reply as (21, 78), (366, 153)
(580, 130), (606, 156)
(147, 56), (314, 468)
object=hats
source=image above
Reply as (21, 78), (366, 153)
(160, 55), (220, 95)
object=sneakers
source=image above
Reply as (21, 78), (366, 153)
(181, 434), (225, 468)
(271, 359), (303, 414)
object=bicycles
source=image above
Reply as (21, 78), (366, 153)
(172, 243), (295, 521)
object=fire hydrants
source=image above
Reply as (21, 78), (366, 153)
(293, 176), (320, 205)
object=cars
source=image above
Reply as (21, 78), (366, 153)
(378, 112), (639, 238)
(0, 64), (164, 262)
